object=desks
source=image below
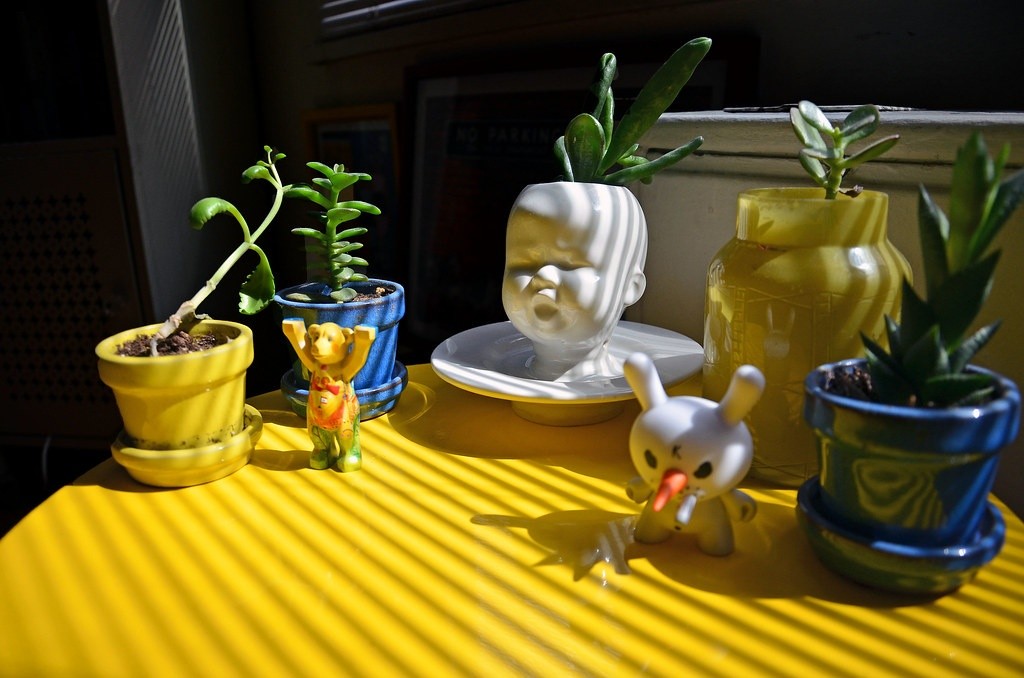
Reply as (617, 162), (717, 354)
(0, 363), (1024, 678)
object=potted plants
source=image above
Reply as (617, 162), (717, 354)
(91, 143), (292, 492)
(792, 132), (1024, 600)
(271, 162), (410, 422)
(430, 37), (713, 431)
(700, 98), (915, 495)
